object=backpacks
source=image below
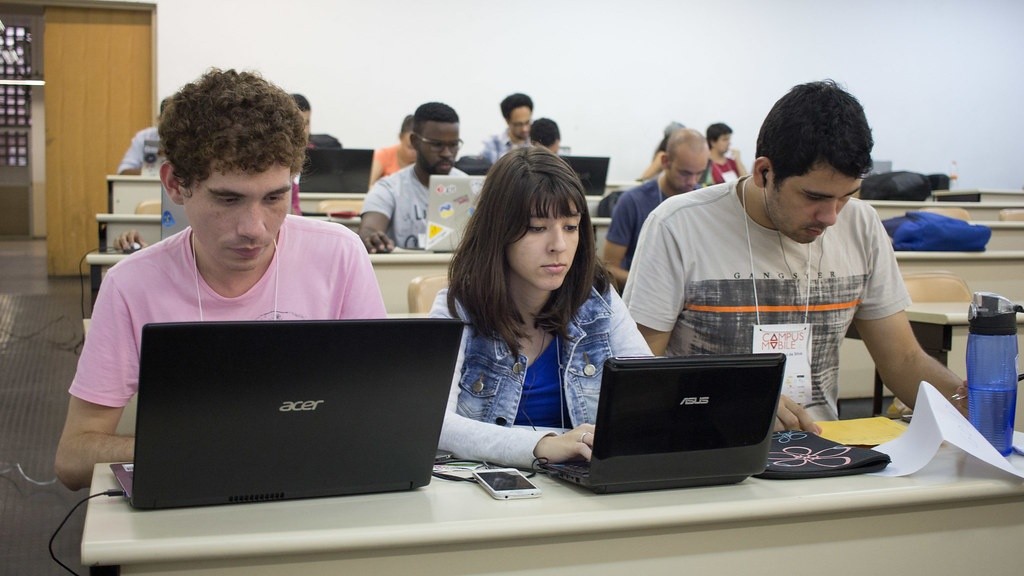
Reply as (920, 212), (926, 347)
(893, 215), (991, 251)
(858, 171), (931, 202)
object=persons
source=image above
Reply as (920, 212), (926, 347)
(358, 102), (468, 253)
(369, 115), (416, 188)
(116, 97), (170, 176)
(602, 128), (709, 292)
(643, 123), (686, 181)
(428, 145), (656, 468)
(529, 118), (568, 155)
(482, 93), (533, 164)
(114, 231), (148, 254)
(698, 122), (747, 188)
(54, 68), (387, 491)
(289, 93), (342, 147)
(623, 80), (969, 434)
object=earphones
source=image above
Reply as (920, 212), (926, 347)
(762, 169), (769, 187)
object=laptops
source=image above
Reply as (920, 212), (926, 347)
(141, 139), (165, 176)
(298, 149), (375, 193)
(160, 183), (189, 240)
(560, 156), (610, 196)
(537, 353), (787, 494)
(110, 318), (465, 509)
(426, 175), (487, 253)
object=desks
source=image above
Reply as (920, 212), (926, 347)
(82, 172), (1024, 576)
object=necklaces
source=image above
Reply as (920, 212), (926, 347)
(193, 233), (279, 320)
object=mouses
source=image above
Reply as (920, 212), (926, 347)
(372, 242), (389, 253)
(123, 241), (142, 254)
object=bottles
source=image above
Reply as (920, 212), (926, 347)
(966, 291), (1019, 458)
(949, 160), (959, 192)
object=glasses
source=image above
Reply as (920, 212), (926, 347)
(413, 132), (463, 151)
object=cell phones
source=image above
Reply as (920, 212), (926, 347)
(472, 467), (542, 500)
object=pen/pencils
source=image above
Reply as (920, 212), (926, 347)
(951, 373), (1024, 401)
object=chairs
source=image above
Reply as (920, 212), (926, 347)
(873, 270), (973, 416)
(1000, 209), (1024, 221)
(408, 273), (451, 314)
(920, 206), (972, 223)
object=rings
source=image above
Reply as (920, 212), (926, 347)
(581, 432), (591, 443)
